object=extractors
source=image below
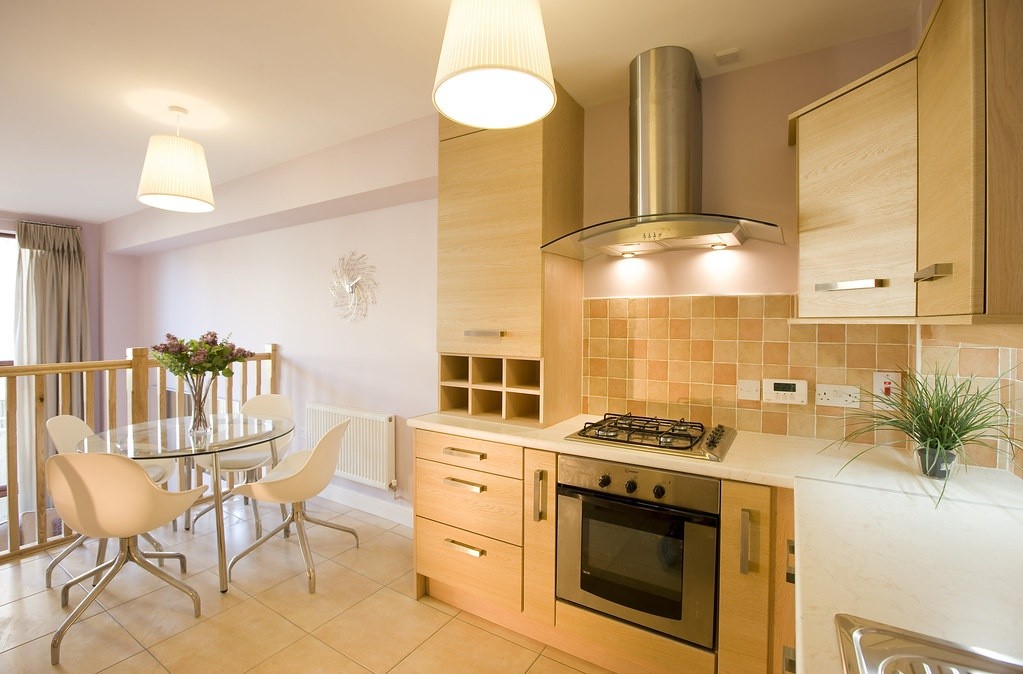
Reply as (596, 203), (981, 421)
(538, 45), (786, 263)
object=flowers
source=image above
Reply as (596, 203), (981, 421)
(150, 330), (256, 378)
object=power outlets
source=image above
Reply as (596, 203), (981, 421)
(737, 380), (760, 401)
(814, 382), (862, 409)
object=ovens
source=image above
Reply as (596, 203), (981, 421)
(555, 453), (721, 649)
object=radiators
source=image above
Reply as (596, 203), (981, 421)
(306, 401), (398, 491)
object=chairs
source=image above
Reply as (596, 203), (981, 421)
(227, 418), (360, 592)
(190, 394), (295, 539)
(44, 449), (209, 668)
(45, 413), (179, 588)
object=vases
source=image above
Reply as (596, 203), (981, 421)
(182, 377), (216, 433)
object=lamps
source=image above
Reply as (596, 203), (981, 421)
(432, 0), (559, 129)
(134, 105), (219, 214)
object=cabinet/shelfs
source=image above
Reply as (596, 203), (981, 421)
(524, 447), (560, 627)
(792, 0), (1023, 324)
(408, 423), (528, 634)
(719, 479), (777, 674)
(434, 120), (585, 428)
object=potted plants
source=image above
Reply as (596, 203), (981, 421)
(827, 363), (1023, 508)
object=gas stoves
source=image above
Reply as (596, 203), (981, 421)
(564, 412), (738, 462)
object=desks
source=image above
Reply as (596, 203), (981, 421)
(75, 414), (295, 595)
